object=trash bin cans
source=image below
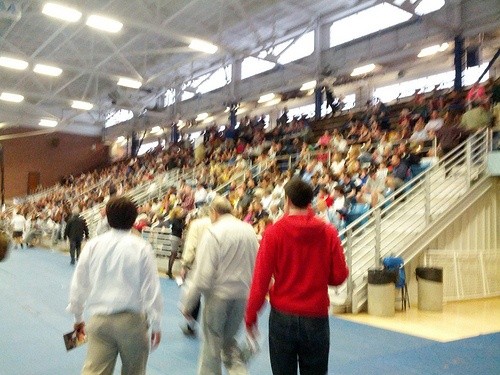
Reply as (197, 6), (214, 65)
(367, 269), (395, 317)
(416, 267), (444, 312)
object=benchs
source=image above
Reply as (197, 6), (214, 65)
(0, 77), (500, 240)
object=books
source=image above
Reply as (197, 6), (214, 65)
(63, 328), (88, 351)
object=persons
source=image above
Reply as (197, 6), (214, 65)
(180, 194), (259, 375)
(180, 203), (213, 337)
(243, 178), (348, 375)
(166, 206), (188, 280)
(11, 209), (26, 249)
(64, 207), (90, 265)
(70, 195), (162, 375)
(2, 74), (500, 248)
